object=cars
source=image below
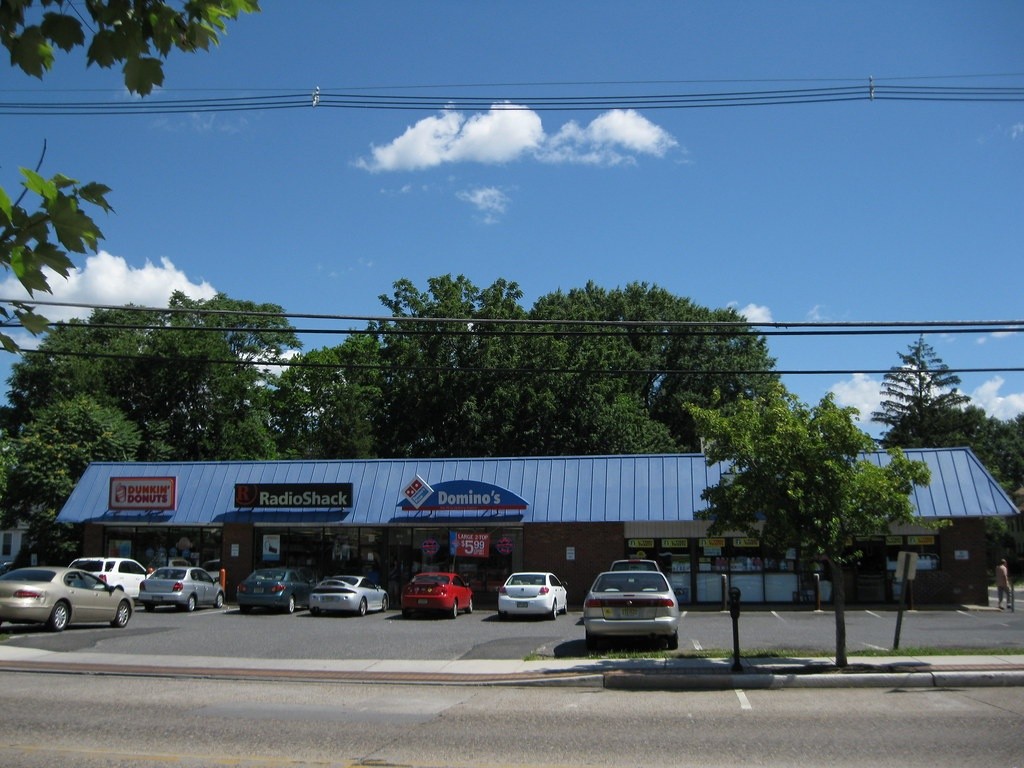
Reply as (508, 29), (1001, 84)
(309, 576), (389, 616)
(137, 566), (225, 612)
(583, 570), (679, 650)
(0, 566), (135, 632)
(68, 555), (149, 599)
(402, 574), (472, 618)
(498, 571), (568, 620)
(236, 567), (310, 614)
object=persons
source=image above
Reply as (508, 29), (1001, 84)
(995, 559), (1011, 610)
(146, 564), (157, 580)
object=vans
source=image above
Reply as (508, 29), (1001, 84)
(611, 559), (662, 573)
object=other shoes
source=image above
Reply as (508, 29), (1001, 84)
(1007, 605), (1012, 608)
(999, 606), (1004, 610)
